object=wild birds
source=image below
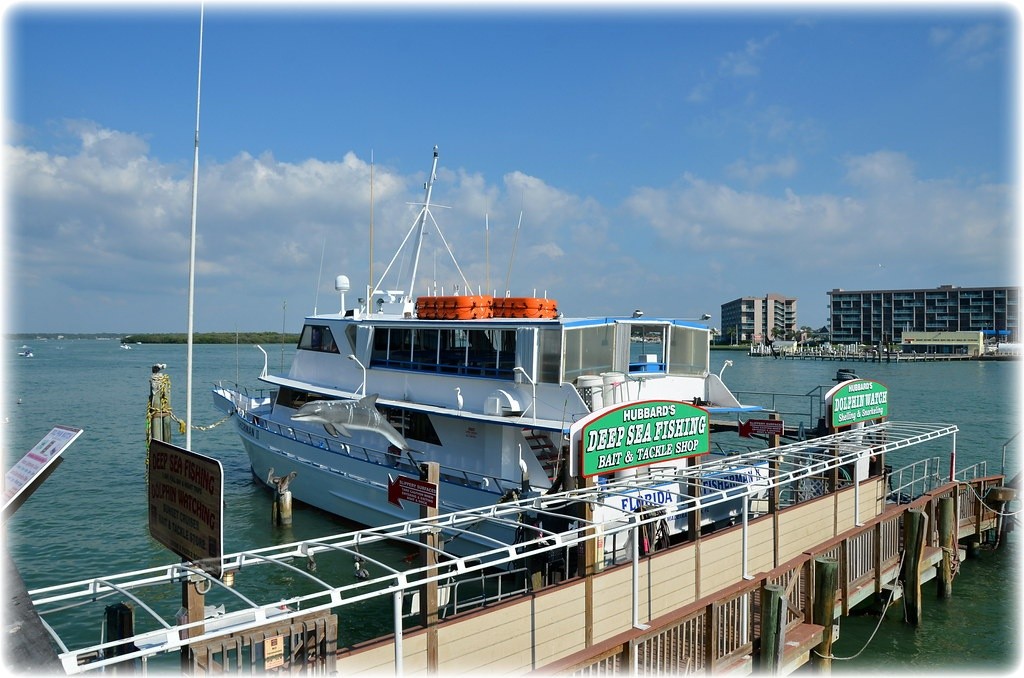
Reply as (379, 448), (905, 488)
(151, 363), (166, 375)
(454, 387), (464, 417)
(517, 443), (528, 474)
(352, 546), (367, 564)
(306, 554), (317, 572)
(353, 562), (370, 579)
(273, 471), (297, 495)
(266, 467), (282, 494)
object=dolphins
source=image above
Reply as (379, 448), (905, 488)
(290, 393), (425, 456)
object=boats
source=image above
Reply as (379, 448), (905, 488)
(199, 142), (782, 578)
